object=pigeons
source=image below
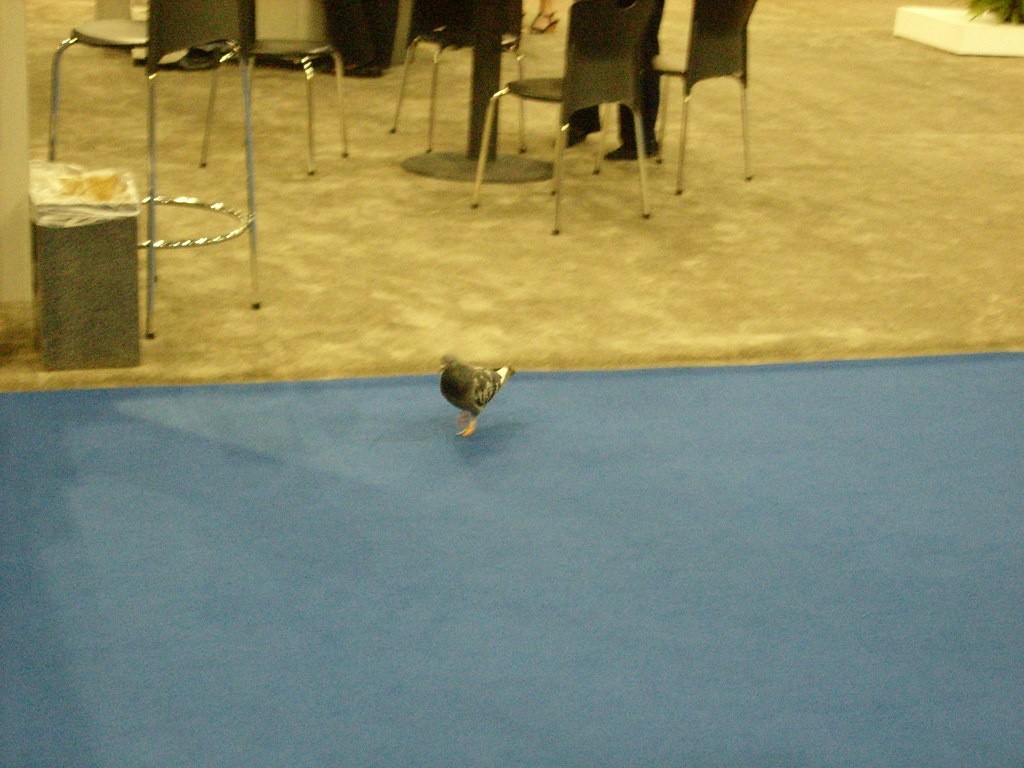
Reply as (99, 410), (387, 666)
(439, 356), (514, 437)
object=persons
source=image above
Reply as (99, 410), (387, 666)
(603, 0), (666, 160)
(529, 0), (560, 34)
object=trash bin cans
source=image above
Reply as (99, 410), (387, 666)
(28, 159), (141, 374)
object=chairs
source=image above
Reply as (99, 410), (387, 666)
(469, 0), (656, 236)
(391, 0), (527, 157)
(594, 0), (757, 193)
(200, 0), (349, 175)
(48, 0), (261, 338)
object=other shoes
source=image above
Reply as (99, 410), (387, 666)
(551, 123), (598, 148)
(605, 137), (659, 160)
